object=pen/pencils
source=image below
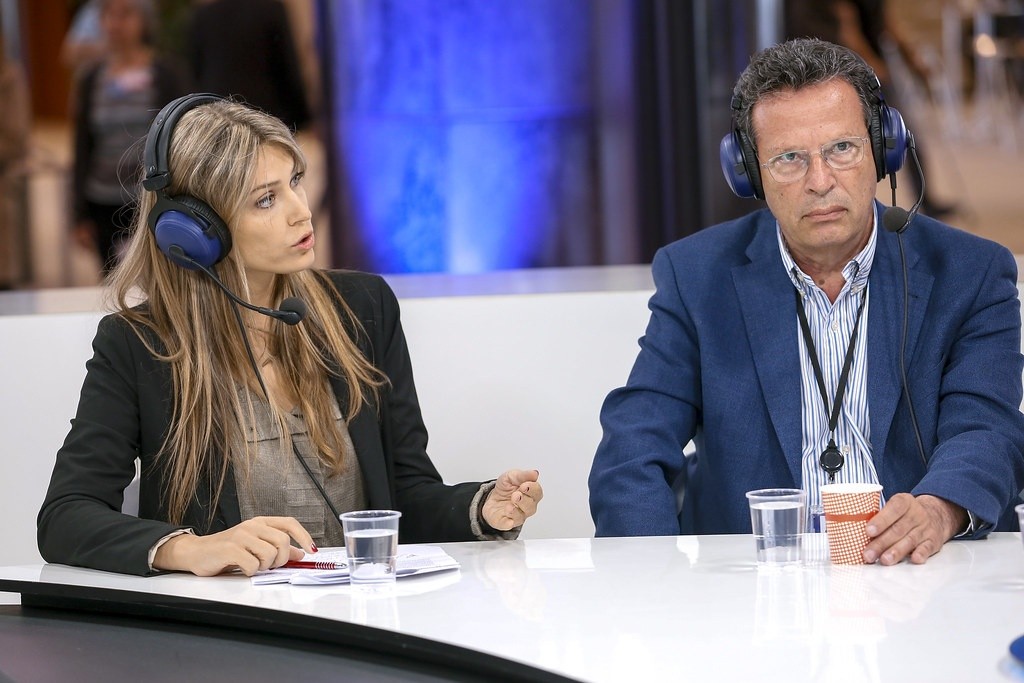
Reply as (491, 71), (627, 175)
(282, 560), (346, 570)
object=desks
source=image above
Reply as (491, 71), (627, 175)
(0, 524), (1024, 683)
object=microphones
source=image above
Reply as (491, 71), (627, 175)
(168, 244), (306, 326)
(882, 128), (926, 235)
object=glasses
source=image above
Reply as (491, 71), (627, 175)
(760, 131), (871, 184)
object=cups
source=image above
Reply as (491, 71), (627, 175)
(1015, 504), (1024, 547)
(818, 483), (884, 564)
(339, 509), (401, 587)
(745, 488), (807, 569)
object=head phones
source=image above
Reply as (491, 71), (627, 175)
(142, 91), (237, 271)
(720, 46), (908, 198)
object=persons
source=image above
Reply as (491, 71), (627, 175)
(63, 2), (195, 285)
(37, 98), (544, 577)
(587, 37), (1023, 567)
